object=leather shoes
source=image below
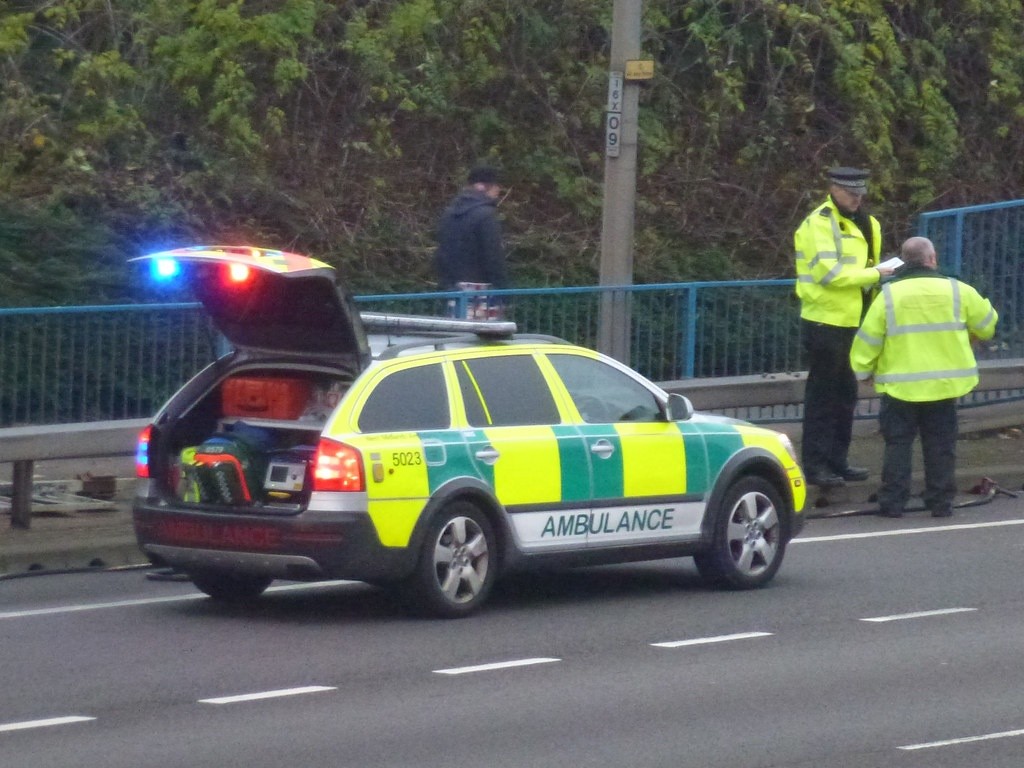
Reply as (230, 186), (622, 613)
(806, 468), (845, 488)
(842, 465), (869, 480)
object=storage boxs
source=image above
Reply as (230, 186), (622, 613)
(220, 375), (307, 423)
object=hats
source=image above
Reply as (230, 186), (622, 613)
(468, 165), (506, 184)
(827, 167), (870, 195)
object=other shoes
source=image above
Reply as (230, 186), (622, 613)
(931, 505), (954, 517)
(882, 506), (902, 517)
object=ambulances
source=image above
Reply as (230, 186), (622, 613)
(126, 240), (812, 619)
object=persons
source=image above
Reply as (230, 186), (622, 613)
(793, 168), (896, 485)
(848, 234), (999, 517)
(435, 167), (512, 322)
